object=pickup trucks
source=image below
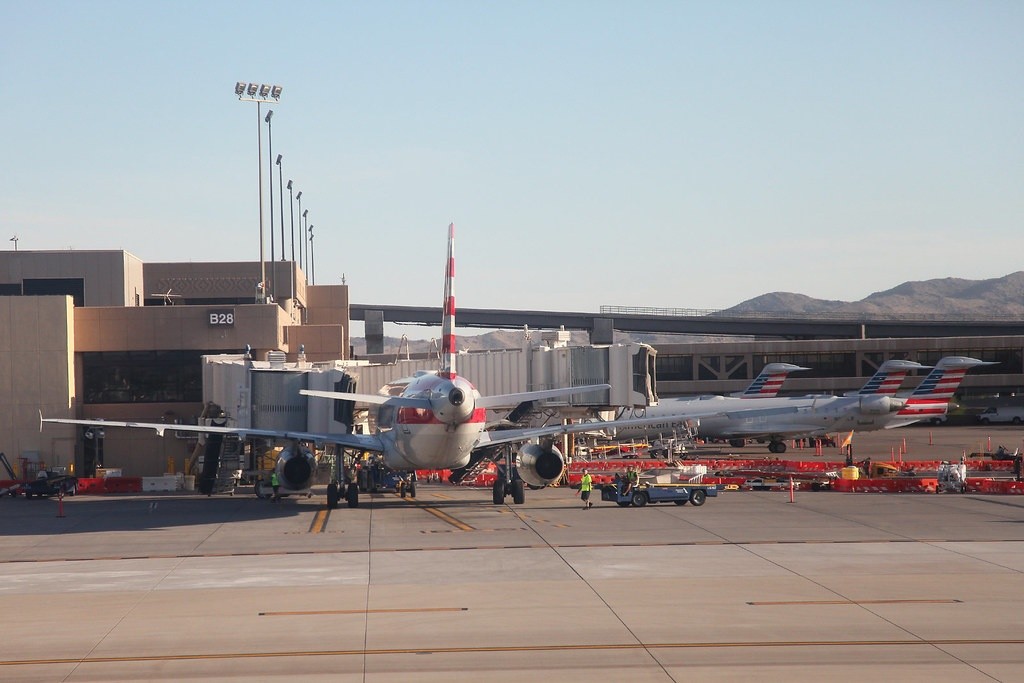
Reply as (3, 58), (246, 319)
(977, 406), (1023, 424)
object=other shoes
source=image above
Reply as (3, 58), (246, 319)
(620, 492), (626, 497)
(583, 506), (588, 510)
(589, 502), (592, 509)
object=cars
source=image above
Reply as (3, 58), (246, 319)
(24, 470), (79, 500)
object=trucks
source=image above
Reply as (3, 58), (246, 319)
(601, 472), (717, 508)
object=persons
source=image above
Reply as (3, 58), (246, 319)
(576, 468), (593, 510)
(270, 467), (281, 498)
(864, 457), (871, 479)
(622, 465), (639, 496)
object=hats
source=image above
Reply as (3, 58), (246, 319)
(582, 468), (589, 473)
(627, 465), (635, 469)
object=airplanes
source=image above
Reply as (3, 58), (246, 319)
(39, 222), (816, 511)
(604, 356), (1003, 454)
